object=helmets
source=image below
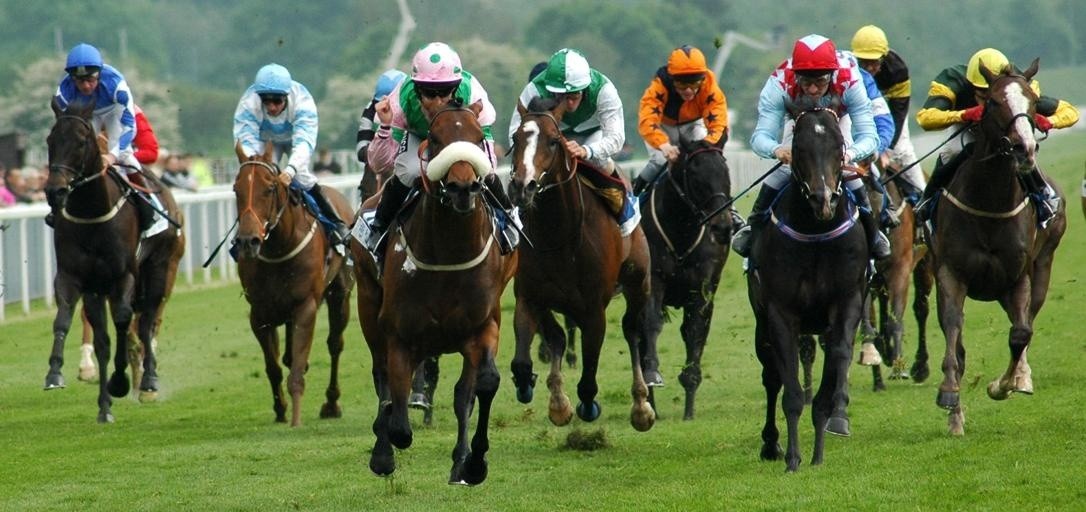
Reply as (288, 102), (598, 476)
(792, 24), (890, 71)
(375, 69), (408, 101)
(667, 45), (707, 77)
(410, 42), (462, 82)
(254, 64), (291, 95)
(966, 48), (1011, 88)
(544, 47), (593, 94)
(64, 43), (104, 78)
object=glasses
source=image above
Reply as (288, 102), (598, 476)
(673, 80), (701, 88)
(554, 93), (581, 100)
(796, 75), (832, 87)
(419, 86), (454, 98)
(261, 96), (282, 105)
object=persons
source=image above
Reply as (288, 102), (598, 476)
(914, 45), (1080, 222)
(311, 147), (342, 176)
(849, 22), (932, 240)
(730, 32), (891, 258)
(0, 145), (215, 208)
(633, 42), (730, 237)
(230, 61), (350, 259)
(129, 103), (158, 166)
(505, 45), (640, 234)
(45, 41), (153, 231)
(834, 49), (897, 223)
(355, 69), (409, 203)
(361, 39), (514, 252)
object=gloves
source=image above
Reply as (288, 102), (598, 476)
(1034, 112), (1054, 131)
(962, 103), (984, 121)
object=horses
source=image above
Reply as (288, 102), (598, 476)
(505, 94), (656, 434)
(43, 83), (185, 429)
(621, 120), (735, 423)
(230, 134), (356, 425)
(910, 55), (1070, 440)
(356, 97), (517, 489)
(738, 106), (936, 472)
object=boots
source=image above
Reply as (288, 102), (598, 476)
(609, 167), (648, 225)
(367, 172), (412, 257)
(127, 169), (163, 233)
(732, 182), (780, 257)
(850, 176), (901, 261)
(1023, 161), (1058, 222)
(912, 154), (944, 221)
(308, 183), (352, 245)
(485, 174), (520, 253)
